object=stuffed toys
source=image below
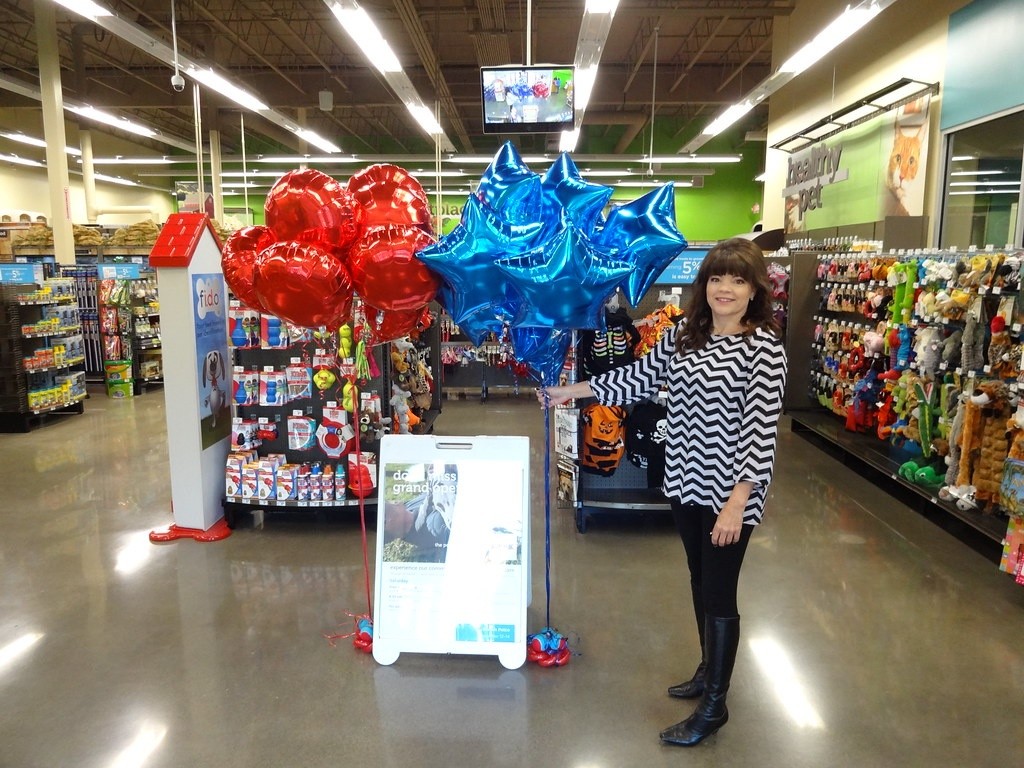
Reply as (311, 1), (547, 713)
(391, 347), (434, 434)
(845, 252), (1024, 511)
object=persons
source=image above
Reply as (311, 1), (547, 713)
(384, 463), (458, 546)
(509, 104), (517, 122)
(553, 76), (561, 94)
(537, 238), (788, 746)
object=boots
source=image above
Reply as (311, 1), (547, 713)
(659, 615), (740, 746)
(667, 615), (706, 698)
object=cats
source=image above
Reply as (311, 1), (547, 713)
(884, 119), (927, 216)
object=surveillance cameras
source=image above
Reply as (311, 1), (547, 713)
(171, 75), (185, 92)
(647, 169), (653, 178)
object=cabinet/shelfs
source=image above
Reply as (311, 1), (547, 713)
(223, 283), (441, 529)
(0, 245), (152, 435)
(565, 248), (708, 533)
(764, 246), (1024, 558)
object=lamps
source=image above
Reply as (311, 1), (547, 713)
(677, 0), (898, 155)
(56, 0), (343, 152)
(324, 0), (458, 153)
(558, 0), (620, 153)
(84, 153), (742, 198)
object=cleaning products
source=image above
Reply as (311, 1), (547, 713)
(298, 461), (347, 501)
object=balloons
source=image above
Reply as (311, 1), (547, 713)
(418, 142), (687, 383)
(221, 164), (442, 345)
(509, 81), (549, 103)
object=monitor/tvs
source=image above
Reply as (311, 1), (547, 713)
(480, 65), (575, 135)
